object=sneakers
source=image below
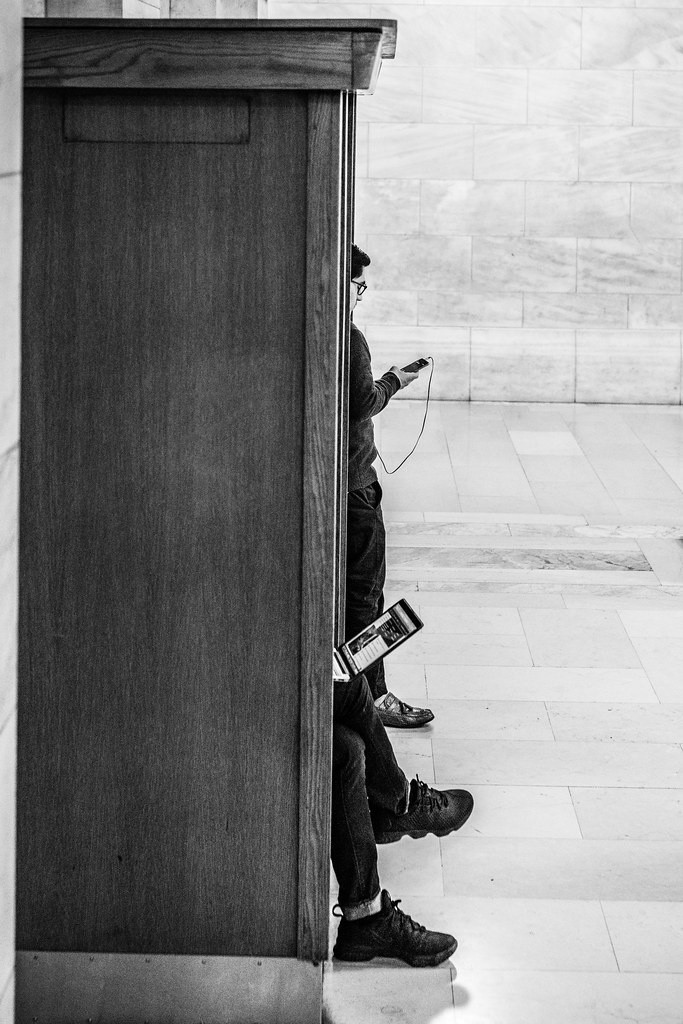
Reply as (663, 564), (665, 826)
(332, 888), (458, 969)
(371, 774), (474, 844)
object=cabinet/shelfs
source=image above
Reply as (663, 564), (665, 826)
(16, 17), (398, 1023)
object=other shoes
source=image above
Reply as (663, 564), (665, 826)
(373, 691), (435, 726)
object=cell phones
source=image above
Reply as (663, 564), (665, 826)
(400, 358), (429, 372)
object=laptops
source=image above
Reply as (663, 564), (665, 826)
(333, 599), (423, 683)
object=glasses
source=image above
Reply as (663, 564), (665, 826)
(351, 280), (367, 295)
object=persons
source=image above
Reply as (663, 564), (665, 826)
(334, 672), (474, 968)
(345, 242), (435, 728)
